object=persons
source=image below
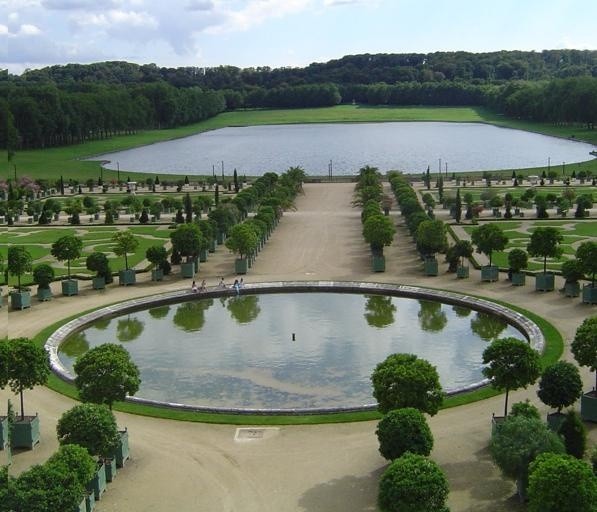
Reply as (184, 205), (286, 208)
(234, 279), (239, 296)
(219, 277), (225, 287)
(201, 280), (207, 292)
(191, 281), (197, 292)
(240, 278), (243, 288)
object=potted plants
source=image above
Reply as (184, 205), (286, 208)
(362, 185), (597, 306)
(476, 316), (597, 506)
(0, 336), (143, 512)
(0, 184), (281, 313)
